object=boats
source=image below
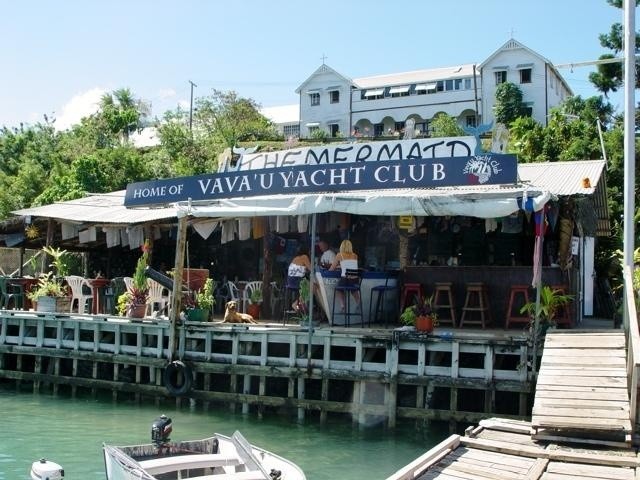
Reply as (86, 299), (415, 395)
(102, 415), (307, 480)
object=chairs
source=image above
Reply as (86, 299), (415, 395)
(66, 275), (165, 325)
(331, 270), (401, 329)
(226, 274), (307, 324)
(0, 275), (22, 311)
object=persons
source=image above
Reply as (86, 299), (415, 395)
(447, 245), (464, 265)
(287, 245), (311, 311)
(318, 240), (337, 266)
(330, 238), (365, 312)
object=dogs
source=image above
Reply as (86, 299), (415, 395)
(222, 301), (258, 324)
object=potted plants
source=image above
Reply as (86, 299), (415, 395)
(118, 238), (153, 321)
(187, 278), (217, 322)
(23, 279), (72, 317)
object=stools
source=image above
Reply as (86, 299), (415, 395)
(400, 283), (574, 331)
